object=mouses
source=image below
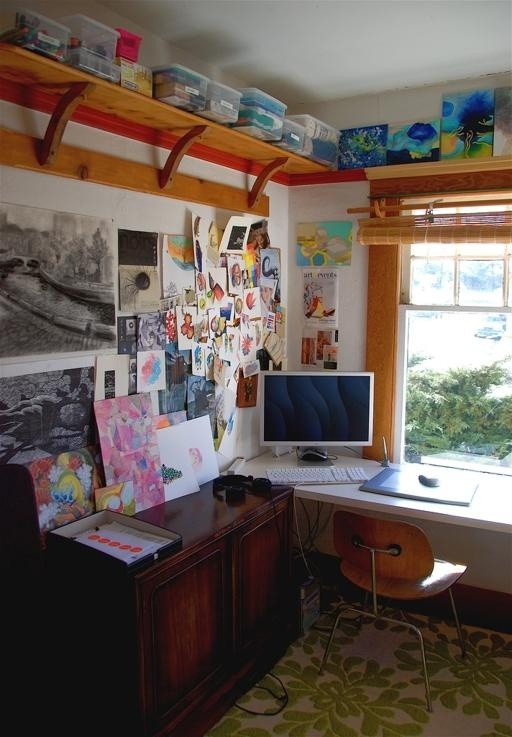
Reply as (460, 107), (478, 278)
(419, 474), (438, 486)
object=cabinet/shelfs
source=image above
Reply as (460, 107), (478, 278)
(47, 488), (301, 736)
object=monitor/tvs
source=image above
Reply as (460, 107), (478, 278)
(258, 370), (374, 467)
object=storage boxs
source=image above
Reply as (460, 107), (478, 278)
(148, 63), (287, 141)
(3, 8), (154, 99)
(271, 114), (341, 168)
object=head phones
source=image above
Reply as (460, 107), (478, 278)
(213, 475), (272, 503)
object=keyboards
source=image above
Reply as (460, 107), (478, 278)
(265, 465), (369, 486)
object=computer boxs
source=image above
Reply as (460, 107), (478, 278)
(291, 546), (321, 637)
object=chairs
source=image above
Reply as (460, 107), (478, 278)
(318, 508), (469, 713)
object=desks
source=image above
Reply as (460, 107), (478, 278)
(238, 451), (512, 532)
